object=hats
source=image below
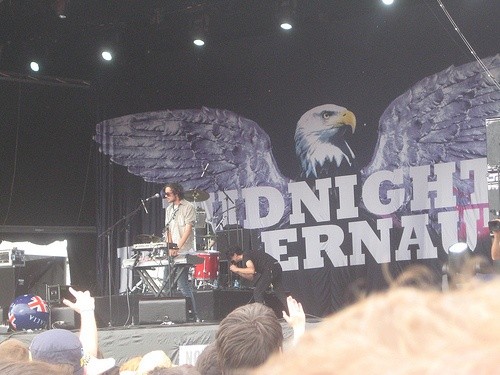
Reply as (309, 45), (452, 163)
(29, 328), (84, 375)
(139, 350), (172, 371)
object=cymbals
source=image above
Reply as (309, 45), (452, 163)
(183, 189), (210, 202)
(138, 234), (164, 242)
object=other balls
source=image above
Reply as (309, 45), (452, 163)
(8, 294), (49, 331)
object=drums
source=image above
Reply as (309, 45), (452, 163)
(193, 250), (220, 280)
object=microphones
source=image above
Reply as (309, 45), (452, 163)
(201, 162), (210, 178)
(145, 193), (160, 201)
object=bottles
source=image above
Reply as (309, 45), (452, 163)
(234, 280), (239, 289)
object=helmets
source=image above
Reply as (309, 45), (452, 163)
(8, 293), (50, 333)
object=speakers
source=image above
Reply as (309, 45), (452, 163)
(138, 296), (196, 325)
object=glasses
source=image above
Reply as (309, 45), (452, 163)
(165, 192), (173, 197)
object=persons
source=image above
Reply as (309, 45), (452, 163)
(488, 220), (500, 260)
(226, 246), (290, 317)
(0, 278), (500, 375)
(164, 182), (200, 323)
(283, 296), (307, 340)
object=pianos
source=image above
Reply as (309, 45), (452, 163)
(121, 253), (204, 270)
(130, 242), (180, 250)
